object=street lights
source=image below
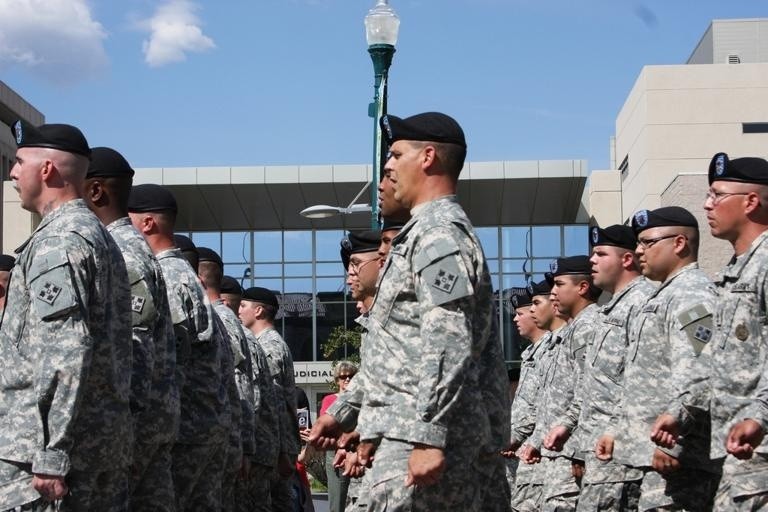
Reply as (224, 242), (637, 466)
(363, 0), (400, 230)
(300, 202), (374, 223)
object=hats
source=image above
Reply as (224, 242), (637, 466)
(375, 209), (412, 233)
(218, 275), (280, 311)
(707, 153), (768, 186)
(339, 229), (382, 272)
(589, 205), (698, 246)
(125, 184), (226, 269)
(510, 256), (592, 308)
(380, 108), (466, 147)
(10, 115), (136, 180)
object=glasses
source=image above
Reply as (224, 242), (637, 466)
(337, 373), (354, 380)
(634, 234), (689, 250)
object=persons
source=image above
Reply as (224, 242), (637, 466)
(300, 113), (513, 512)
(0, 117), (313, 510)
(501, 152), (768, 512)
